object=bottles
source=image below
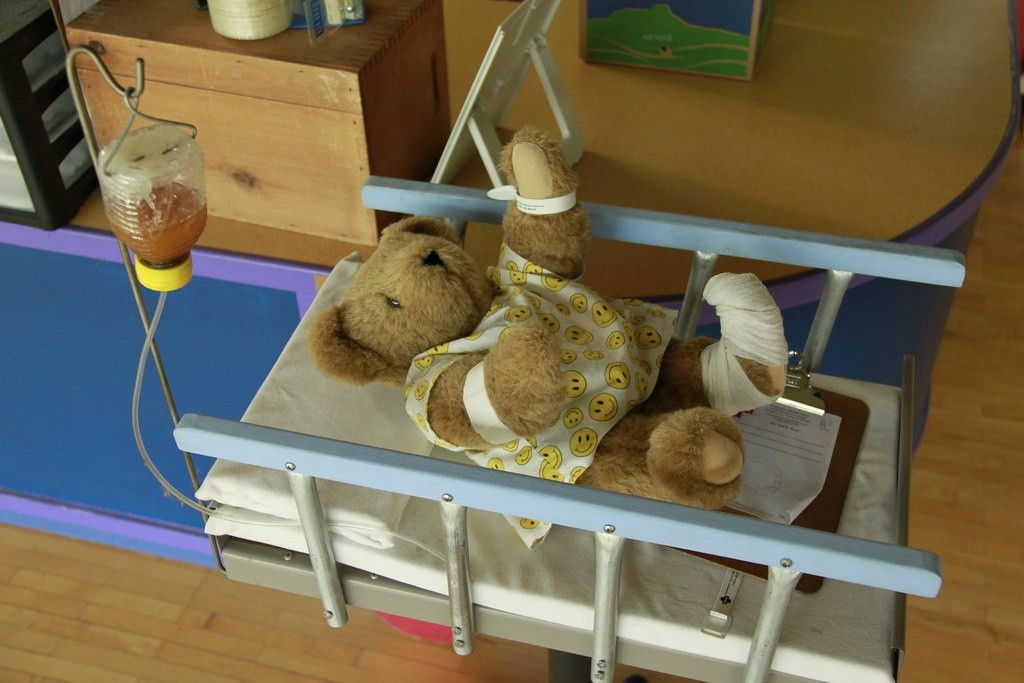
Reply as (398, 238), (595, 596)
(98, 124), (208, 292)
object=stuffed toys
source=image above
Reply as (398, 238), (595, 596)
(310, 126), (787, 549)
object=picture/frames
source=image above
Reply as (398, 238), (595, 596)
(431, 0), (585, 190)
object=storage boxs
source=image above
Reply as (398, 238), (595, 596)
(579, 0), (776, 81)
(64, 0), (450, 247)
